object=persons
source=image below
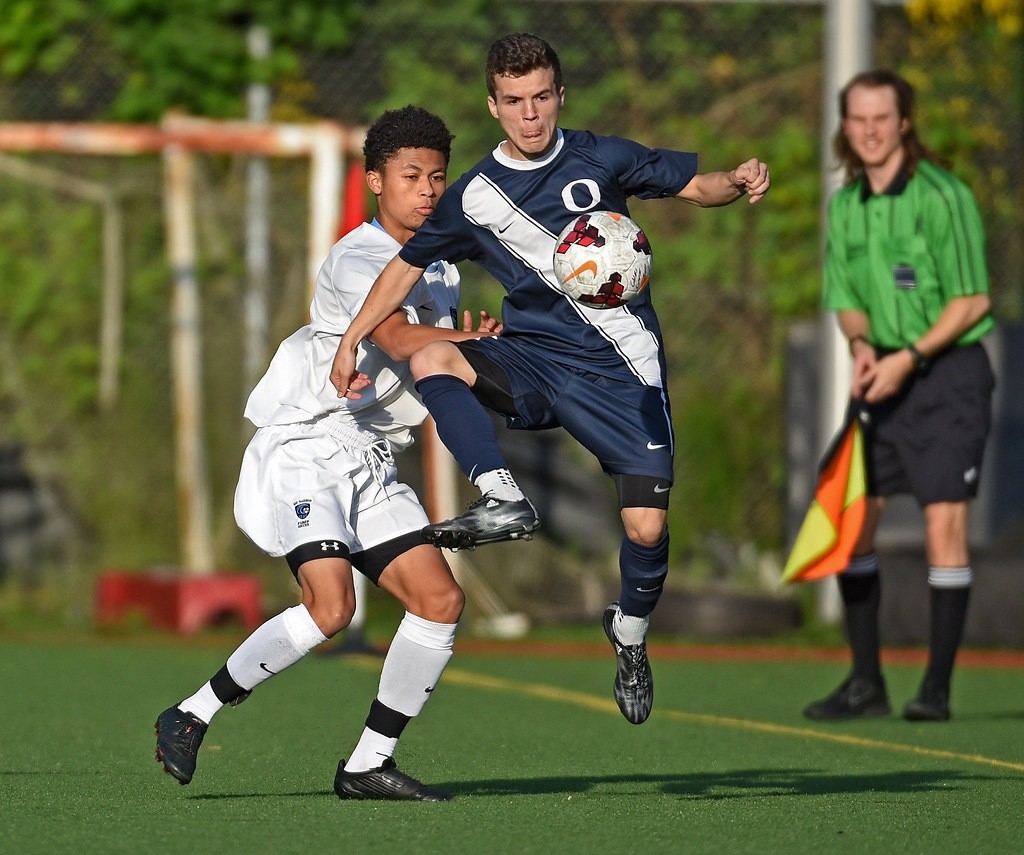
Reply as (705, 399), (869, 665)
(798, 69), (997, 724)
(328, 34), (769, 727)
(155, 101), (502, 800)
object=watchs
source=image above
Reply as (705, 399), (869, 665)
(906, 342), (931, 371)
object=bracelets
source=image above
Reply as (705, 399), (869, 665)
(850, 335), (875, 358)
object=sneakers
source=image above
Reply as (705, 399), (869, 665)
(420, 495), (541, 552)
(154, 703), (207, 786)
(804, 674), (889, 721)
(903, 695), (951, 720)
(333, 758), (447, 802)
(602, 600), (654, 725)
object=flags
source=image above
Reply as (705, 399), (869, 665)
(781, 417), (868, 584)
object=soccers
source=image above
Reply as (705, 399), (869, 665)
(553, 211), (653, 310)
